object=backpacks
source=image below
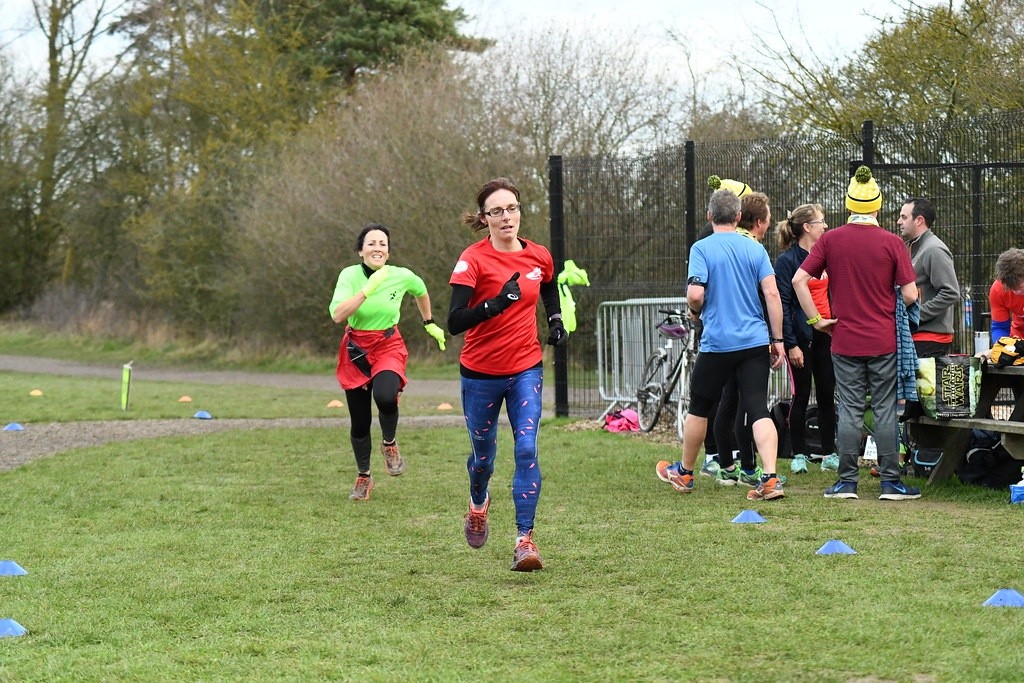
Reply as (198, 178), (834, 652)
(954, 428), (1024, 489)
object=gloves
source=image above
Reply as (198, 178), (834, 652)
(424, 323), (446, 351)
(482, 272), (522, 319)
(362, 264), (392, 297)
(546, 318), (568, 348)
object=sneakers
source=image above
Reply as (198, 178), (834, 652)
(656, 460), (693, 493)
(715, 464), (742, 487)
(350, 477), (375, 500)
(820, 453), (840, 473)
(790, 454), (808, 474)
(699, 460), (719, 478)
(823, 480), (859, 500)
(380, 442), (405, 476)
(747, 478), (785, 501)
(511, 530), (543, 572)
(879, 481), (921, 501)
(464, 490), (490, 549)
(737, 468), (787, 488)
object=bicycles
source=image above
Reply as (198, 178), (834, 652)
(633, 309), (701, 442)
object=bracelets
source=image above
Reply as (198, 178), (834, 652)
(805, 313), (821, 325)
(772, 338), (784, 343)
(424, 316), (434, 325)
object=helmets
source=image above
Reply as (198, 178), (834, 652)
(656, 323), (688, 339)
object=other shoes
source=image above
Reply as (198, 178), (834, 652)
(870, 461), (908, 477)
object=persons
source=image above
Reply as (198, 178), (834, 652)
(447, 177), (569, 572)
(329, 224), (446, 502)
(773, 204), (840, 473)
(698, 176), (753, 478)
(897, 198), (960, 353)
(655, 187), (786, 501)
(792, 165), (922, 501)
(714, 192), (787, 487)
(989, 248), (1024, 346)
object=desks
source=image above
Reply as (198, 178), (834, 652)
(926, 365), (1024, 488)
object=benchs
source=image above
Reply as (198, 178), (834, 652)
(905, 416), (1024, 449)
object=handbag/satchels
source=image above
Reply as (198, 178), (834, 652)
(915, 354), (989, 421)
(766, 353), (795, 412)
(911, 448), (953, 481)
(991, 335), (1024, 370)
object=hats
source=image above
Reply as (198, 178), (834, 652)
(707, 175), (752, 200)
(845, 165), (882, 214)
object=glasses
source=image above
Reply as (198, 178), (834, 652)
(481, 205), (520, 217)
(800, 217), (825, 225)
